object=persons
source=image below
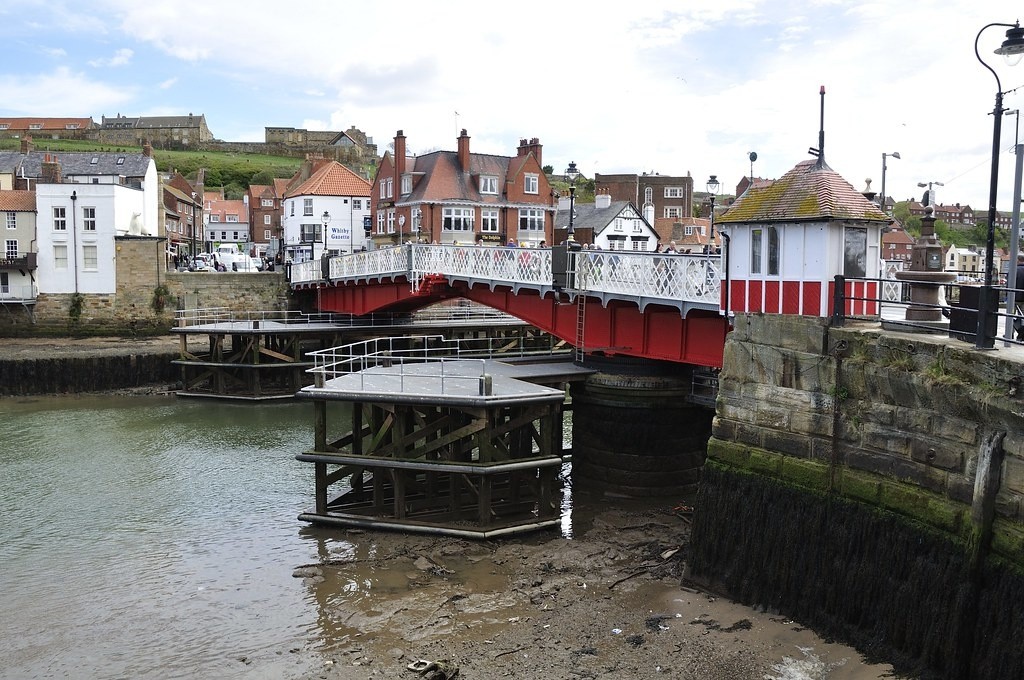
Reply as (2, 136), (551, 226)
(173, 250), (238, 273)
(405, 235), (717, 296)
(261, 257), (274, 271)
(1004, 254), (1024, 342)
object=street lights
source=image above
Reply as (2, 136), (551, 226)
(919, 180), (946, 216)
(881, 152), (899, 209)
(321, 210), (330, 254)
(973, 18), (1024, 352)
(707, 175), (719, 242)
(565, 160), (581, 242)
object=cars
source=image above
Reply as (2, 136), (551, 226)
(252, 256), (265, 272)
(192, 250), (217, 272)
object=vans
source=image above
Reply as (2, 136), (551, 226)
(215, 252), (259, 274)
(216, 243), (239, 253)
